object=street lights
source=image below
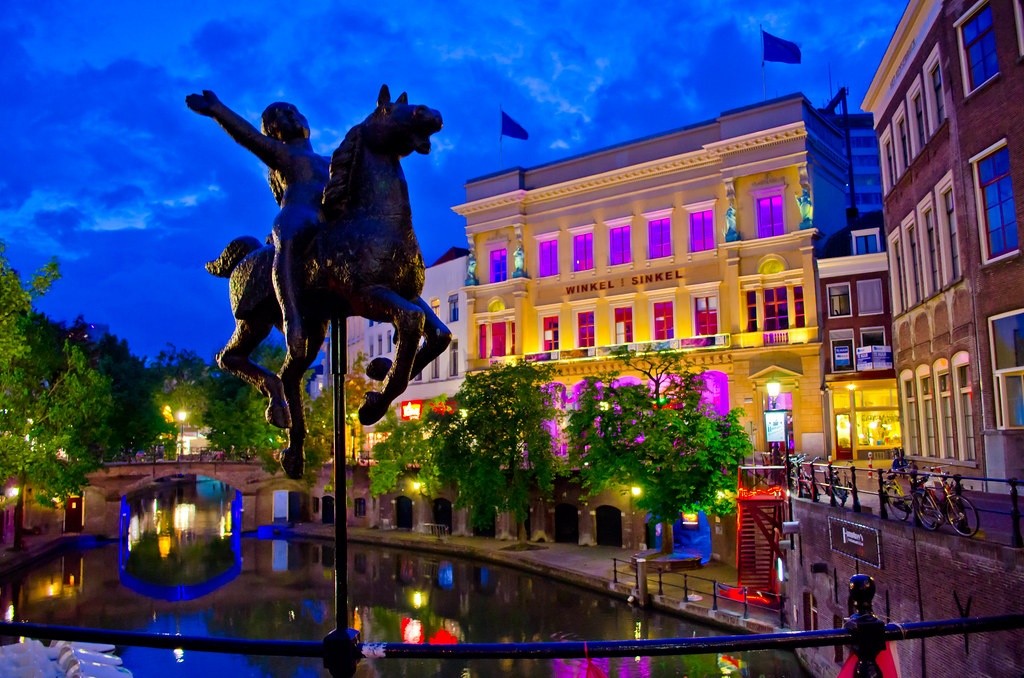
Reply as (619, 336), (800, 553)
(178, 410), (186, 460)
(764, 376), (781, 485)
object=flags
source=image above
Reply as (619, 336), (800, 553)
(761, 29), (801, 64)
(500, 110), (529, 141)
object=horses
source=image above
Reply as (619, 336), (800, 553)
(202, 82), (454, 430)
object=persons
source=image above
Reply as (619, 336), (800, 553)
(794, 187), (813, 224)
(184, 90), (333, 356)
(725, 203), (737, 234)
(512, 246), (525, 273)
(465, 253), (476, 280)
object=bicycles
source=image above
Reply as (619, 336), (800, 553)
(781, 451), (980, 537)
